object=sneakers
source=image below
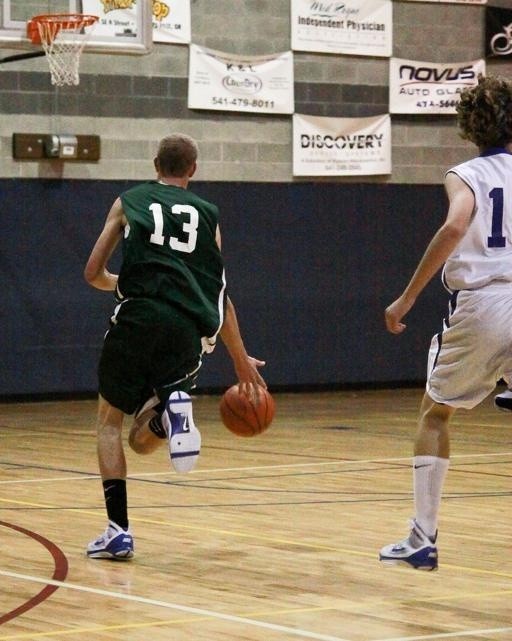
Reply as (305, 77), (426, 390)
(161, 391), (201, 474)
(379, 520), (438, 570)
(87, 519), (133, 559)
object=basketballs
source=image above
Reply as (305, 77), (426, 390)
(220, 382), (275, 438)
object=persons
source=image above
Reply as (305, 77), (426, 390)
(378, 75), (510, 572)
(83, 133), (269, 562)
